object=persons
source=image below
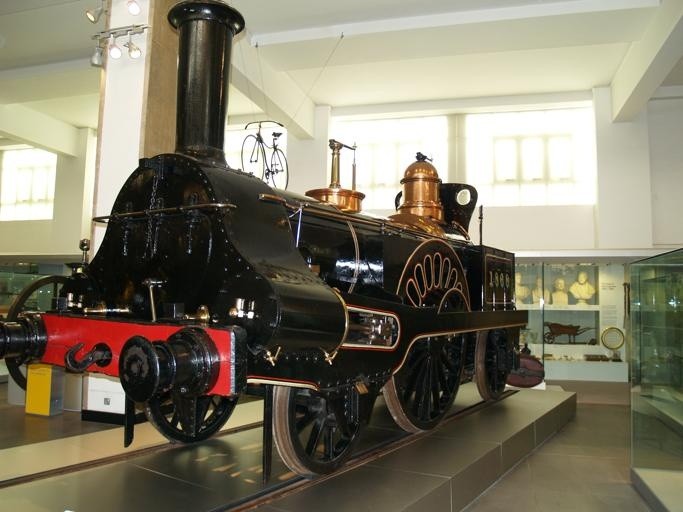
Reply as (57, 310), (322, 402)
(551, 278), (568, 305)
(569, 271), (596, 300)
(532, 276), (550, 304)
(514, 272), (530, 299)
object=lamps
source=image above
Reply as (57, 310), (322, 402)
(90, 36), (102, 68)
(84, 5), (106, 24)
(126, 30), (142, 58)
(108, 34), (122, 60)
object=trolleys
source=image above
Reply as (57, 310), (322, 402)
(540, 321), (592, 342)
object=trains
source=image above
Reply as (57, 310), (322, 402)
(0, 1), (543, 478)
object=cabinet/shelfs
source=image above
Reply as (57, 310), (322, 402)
(630, 249), (683, 511)
(516, 249), (660, 405)
